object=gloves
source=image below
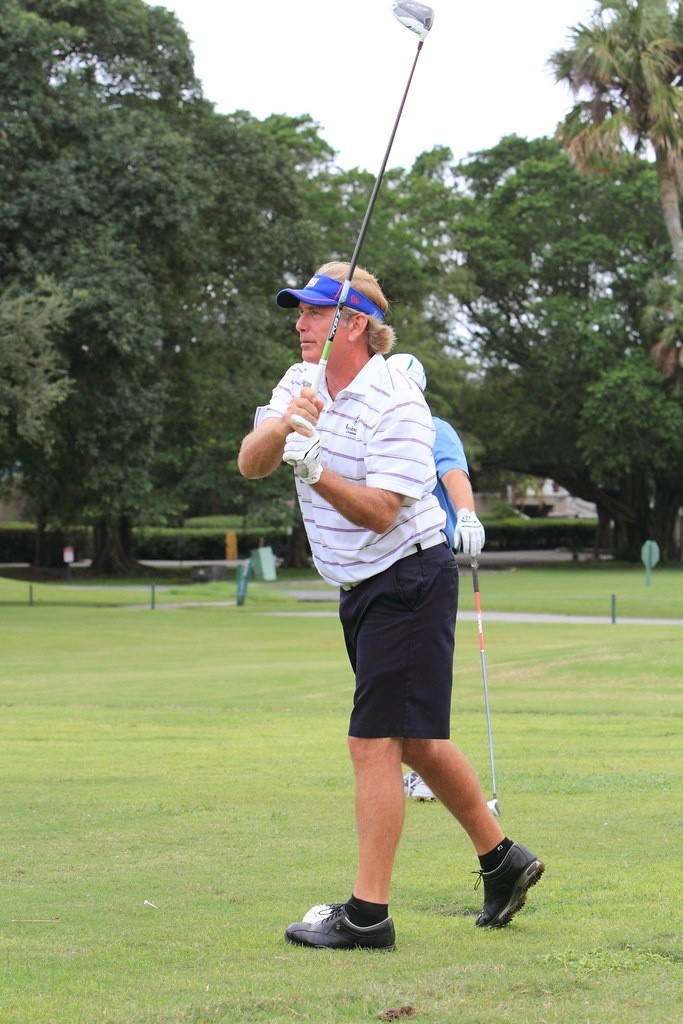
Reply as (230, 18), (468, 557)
(282, 413), (323, 485)
(454, 508), (486, 558)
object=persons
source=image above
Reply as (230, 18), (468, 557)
(237, 259), (551, 952)
(383, 353), (485, 801)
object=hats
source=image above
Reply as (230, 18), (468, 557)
(275, 276), (385, 324)
(387, 354), (427, 392)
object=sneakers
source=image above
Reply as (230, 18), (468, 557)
(472, 841), (546, 928)
(404, 771), (436, 801)
(282, 903), (397, 954)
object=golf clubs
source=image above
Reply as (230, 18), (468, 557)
(279, 0), (440, 463)
(470, 555), (501, 817)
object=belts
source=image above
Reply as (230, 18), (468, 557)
(402, 529), (448, 558)
(342, 582), (360, 592)
